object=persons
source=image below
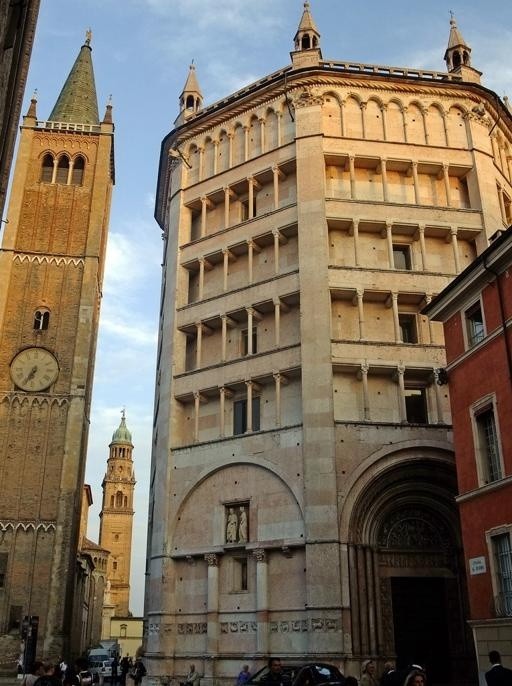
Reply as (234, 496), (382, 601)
(14, 649), (147, 686)
(360, 659), (427, 685)
(260, 657), (359, 685)
(186, 663), (200, 686)
(236, 663), (253, 686)
(238, 506), (248, 544)
(484, 650), (512, 686)
(227, 508), (238, 544)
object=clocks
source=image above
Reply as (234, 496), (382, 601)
(8, 348), (59, 393)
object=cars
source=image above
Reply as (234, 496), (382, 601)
(234, 663), (360, 686)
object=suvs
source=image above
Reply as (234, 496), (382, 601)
(85, 659), (122, 681)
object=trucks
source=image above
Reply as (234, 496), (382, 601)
(85, 638), (121, 672)
